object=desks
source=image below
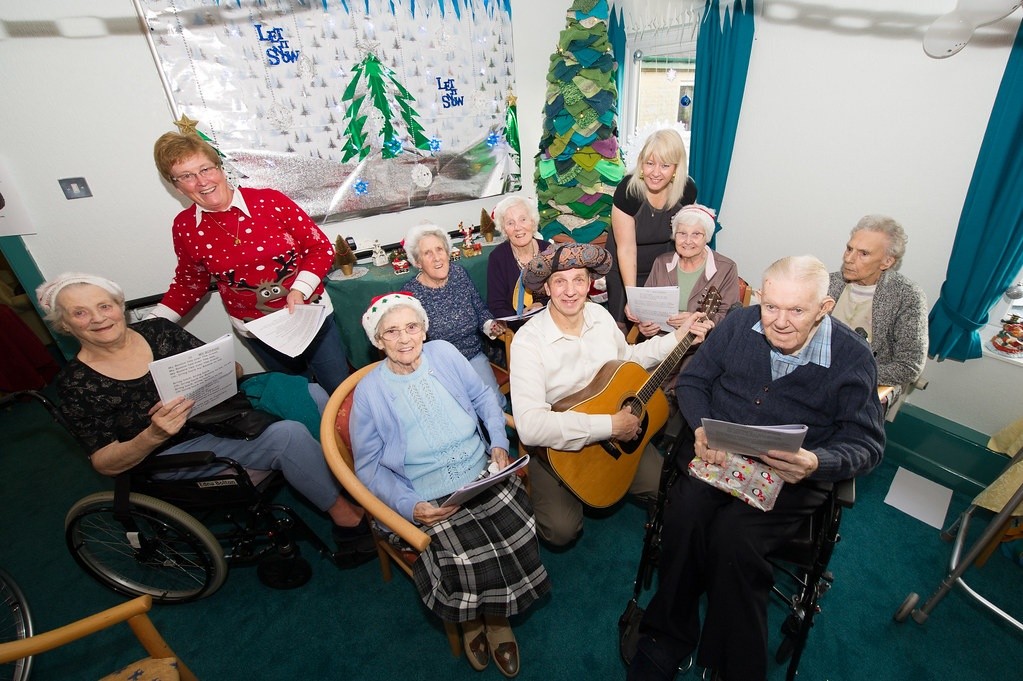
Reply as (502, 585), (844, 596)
(320, 231), (509, 370)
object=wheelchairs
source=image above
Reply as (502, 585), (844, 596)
(0, 568), (38, 681)
(1, 388), (379, 606)
(613, 405), (857, 681)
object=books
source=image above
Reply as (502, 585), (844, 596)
(700, 418), (808, 458)
(149, 333), (237, 420)
(441, 454), (530, 509)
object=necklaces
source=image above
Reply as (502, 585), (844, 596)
(206, 213), (241, 245)
(515, 243), (536, 270)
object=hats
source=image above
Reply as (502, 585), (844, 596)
(521, 240), (613, 298)
(362, 292), (429, 350)
(35, 273), (125, 333)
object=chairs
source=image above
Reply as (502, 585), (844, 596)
(625, 275), (753, 346)
(0, 591), (201, 681)
(480, 326), (513, 397)
(319, 363), (526, 654)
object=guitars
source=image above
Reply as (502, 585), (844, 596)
(536, 284), (721, 510)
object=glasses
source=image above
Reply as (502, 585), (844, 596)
(675, 232), (705, 241)
(378, 322), (423, 341)
(171, 164), (219, 184)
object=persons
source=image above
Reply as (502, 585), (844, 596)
(639, 204), (740, 337)
(349, 292), (551, 678)
(36, 272), (370, 541)
(627, 255), (887, 681)
(510, 243), (715, 545)
(486, 195), (552, 330)
(604, 129), (697, 338)
(829, 215), (929, 417)
(399, 225), (507, 413)
(143, 132), (349, 397)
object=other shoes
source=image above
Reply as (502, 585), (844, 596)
(331, 512), (377, 553)
(627, 633), (694, 681)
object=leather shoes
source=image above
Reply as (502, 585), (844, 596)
(485, 615), (520, 679)
(459, 615), (490, 671)
